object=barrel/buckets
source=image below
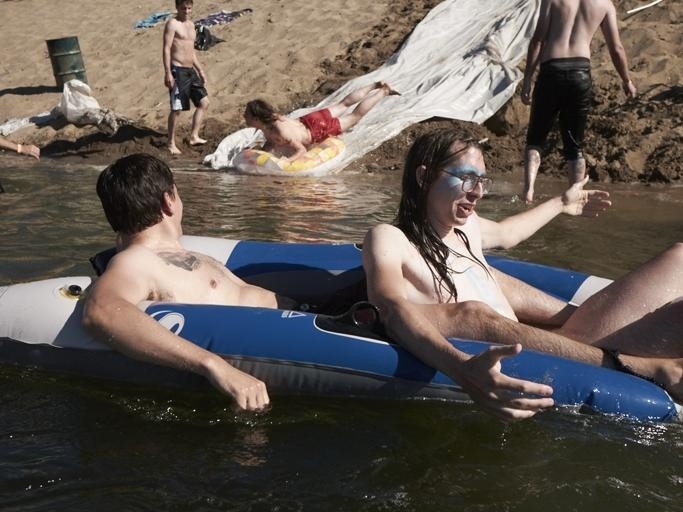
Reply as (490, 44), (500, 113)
(44, 36), (88, 87)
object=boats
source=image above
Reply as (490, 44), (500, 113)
(0, 235), (682, 426)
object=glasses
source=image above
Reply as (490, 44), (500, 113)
(440, 168), (494, 195)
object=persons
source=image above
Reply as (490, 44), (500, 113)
(244, 80), (402, 163)
(359, 117), (682, 425)
(520, 0), (638, 205)
(80, 151), (682, 416)
(161, 0), (211, 155)
